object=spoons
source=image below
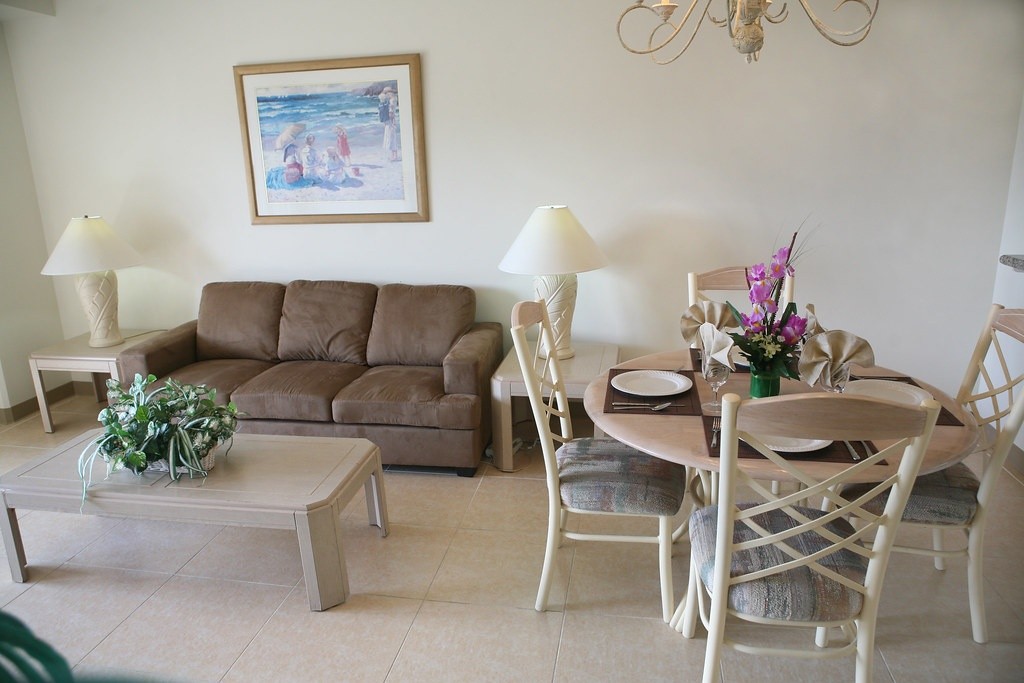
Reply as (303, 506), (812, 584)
(614, 402), (671, 411)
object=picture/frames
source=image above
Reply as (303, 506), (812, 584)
(233, 53), (431, 227)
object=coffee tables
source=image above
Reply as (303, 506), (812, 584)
(489, 341), (622, 474)
(27, 327), (167, 434)
(0, 427), (391, 614)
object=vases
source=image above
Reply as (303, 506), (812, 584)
(748, 363), (780, 399)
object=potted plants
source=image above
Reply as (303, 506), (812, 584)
(76, 371), (251, 518)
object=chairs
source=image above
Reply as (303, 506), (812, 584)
(686, 265), (795, 495)
(509, 296), (687, 624)
(834, 304), (1024, 643)
(682, 393), (941, 683)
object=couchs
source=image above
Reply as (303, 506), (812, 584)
(108, 280), (503, 479)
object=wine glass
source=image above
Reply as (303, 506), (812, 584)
(701, 350), (731, 412)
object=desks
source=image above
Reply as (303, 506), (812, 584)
(583, 343), (982, 642)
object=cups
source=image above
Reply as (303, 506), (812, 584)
(819, 359), (851, 394)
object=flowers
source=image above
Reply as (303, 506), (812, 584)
(725, 212), (809, 381)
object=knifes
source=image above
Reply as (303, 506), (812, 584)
(611, 402), (686, 406)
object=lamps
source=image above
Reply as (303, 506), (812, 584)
(497, 204), (606, 360)
(40, 214), (145, 349)
(615, 0), (879, 65)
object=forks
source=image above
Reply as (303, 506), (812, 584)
(710, 418), (721, 448)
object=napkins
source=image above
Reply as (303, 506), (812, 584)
(680, 300), (739, 381)
(797, 304), (876, 390)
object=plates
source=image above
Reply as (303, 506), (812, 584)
(729, 345), (793, 367)
(842, 379), (934, 405)
(738, 432), (833, 452)
(611, 370), (693, 397)
